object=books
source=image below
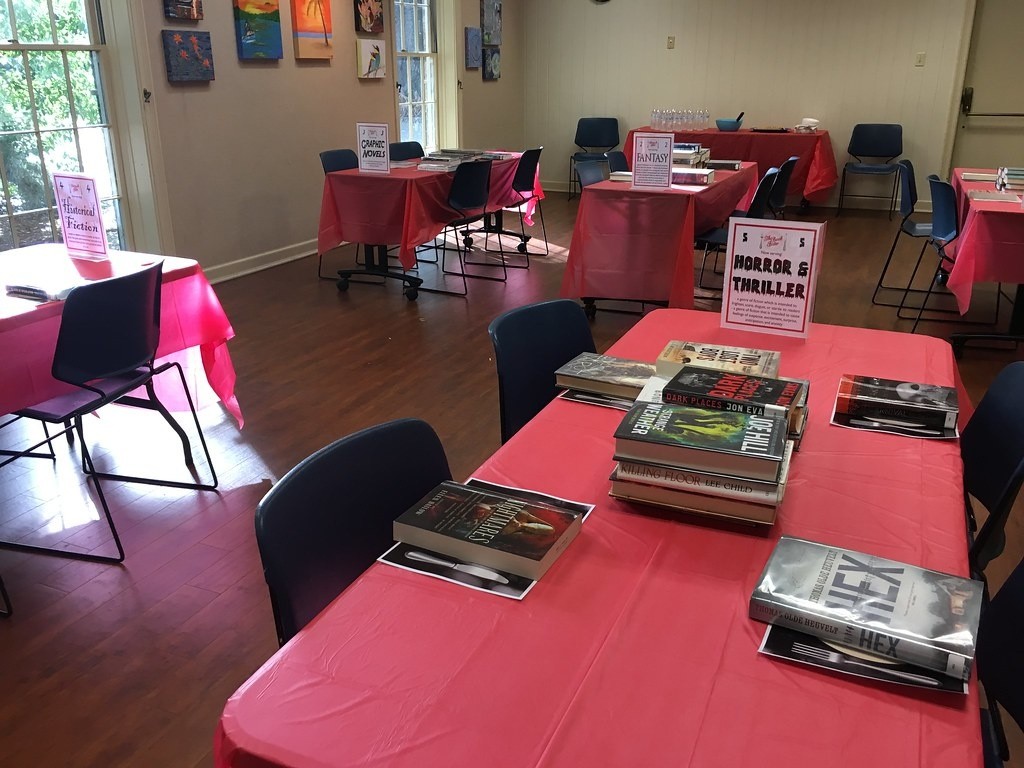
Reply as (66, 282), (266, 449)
(6, 274), (95, 301)
(418, 149), (512, 172)
(748, 534), (984, 682)
(672, 143), (710, 169)
(609, 339), (811, 526)
(672, 168), (714, 185)
(554, 352), (656, 401)
(997, 167), (1024, 195)
(608, 171), (632, 182)
(707, 160), (741, 170)
(394, 480), (582, 582)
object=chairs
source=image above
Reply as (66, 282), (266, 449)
(0, 118), (1024, 768)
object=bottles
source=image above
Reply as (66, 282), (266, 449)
(651, 108), (710, 132)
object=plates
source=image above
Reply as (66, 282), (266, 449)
(751, 127), (791, 132)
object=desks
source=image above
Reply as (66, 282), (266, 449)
(318, 150), (543, 301)
(621, 126), (838, 214)
(558, 159), (759, 318)
(936, 167), (1024, 359)
(217, 308), (982, 768)
(0, 242), (244, 430)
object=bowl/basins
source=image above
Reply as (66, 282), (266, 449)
(794, 118), (820, 133)
(715, 119), (743, 132)
